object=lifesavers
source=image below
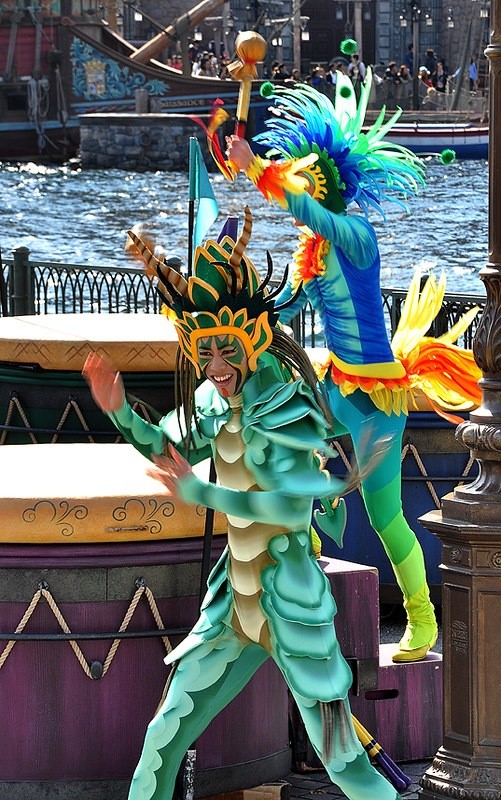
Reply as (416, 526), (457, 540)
(139, 132), (152, 147)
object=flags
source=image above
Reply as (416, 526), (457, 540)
(192, 147), (219, 249)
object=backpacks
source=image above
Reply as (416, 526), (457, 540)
(350, 61), (363, 82)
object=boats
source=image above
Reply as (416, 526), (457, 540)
(50, 0), (491, 163)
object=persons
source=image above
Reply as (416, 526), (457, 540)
(81, 207), (397, 799)
(166, 44), (481, 109)
(224, 42), (483, 661)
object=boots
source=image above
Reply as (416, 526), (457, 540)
(392, 585), (438, 661)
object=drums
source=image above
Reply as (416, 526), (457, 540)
(0, 309), (192, 446)
(0, 438), (296, 800)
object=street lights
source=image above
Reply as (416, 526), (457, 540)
(398, 1), (433, 108)
